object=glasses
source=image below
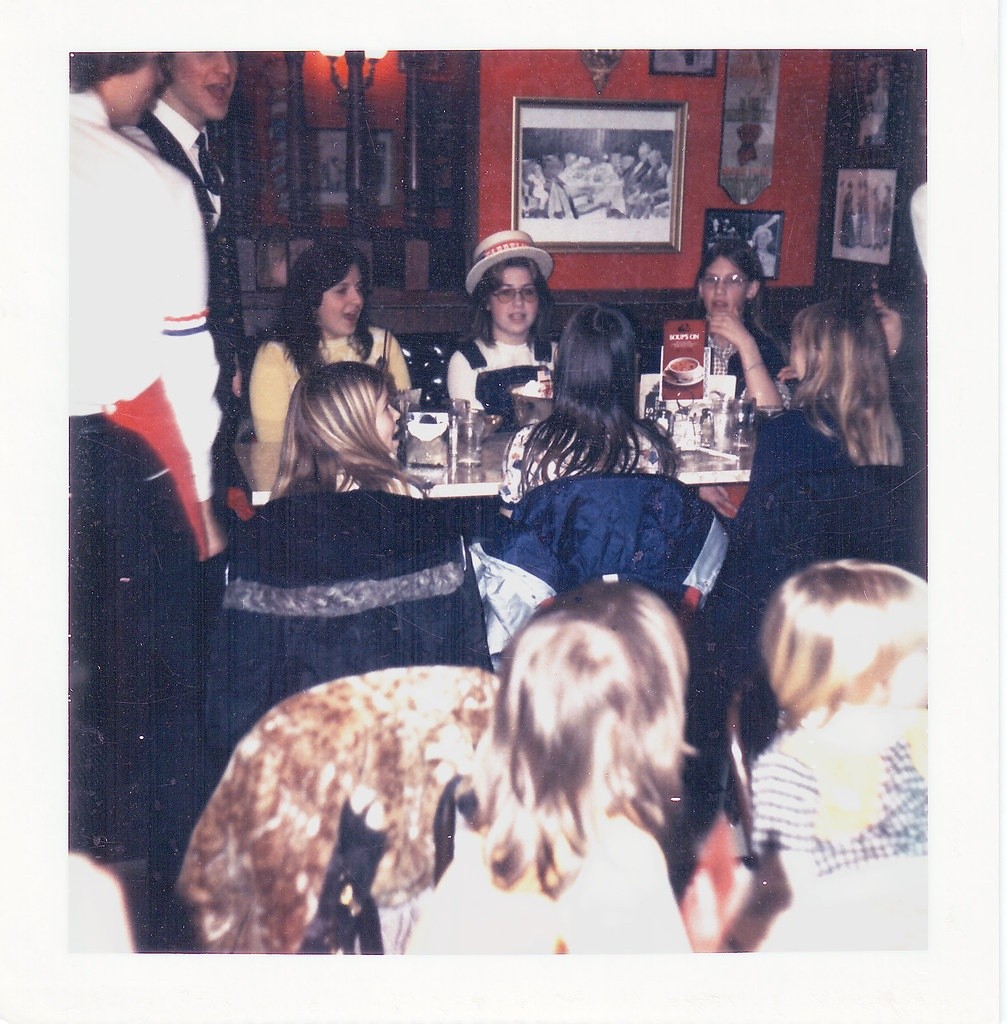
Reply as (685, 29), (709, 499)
(700, 273), (750, 288)
(488, 283), (543, 303)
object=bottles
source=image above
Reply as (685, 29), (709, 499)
(699, 409), (715, 447)
(655, 410), (673, 437)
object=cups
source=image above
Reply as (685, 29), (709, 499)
(453, 408), (486, 469)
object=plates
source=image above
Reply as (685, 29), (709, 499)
(662, 365), (704, 386)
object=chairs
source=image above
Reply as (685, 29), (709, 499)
(179, 465), (928, 949)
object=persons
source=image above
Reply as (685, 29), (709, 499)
(270, 361), (431, 501)
(521, 141), (671, 221)
(69, 51), (251, 954)
(446, 230), (562, 430)
(719, 218), (736, 236)
(750, 225), (775, 277)
(251, 242), (413, 444)
(375, 581), (754, 955)
(497, 305), (680, 518)
(744, 561), (928, 956)
(686, 240), (797, 412)
(696, 280), (929, 526)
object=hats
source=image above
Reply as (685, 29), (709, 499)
(465, 231), (555, 297)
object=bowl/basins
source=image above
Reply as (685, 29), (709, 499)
(507, 383), (554, 428)
(668, 357), (700, 382)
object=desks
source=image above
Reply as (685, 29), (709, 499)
(226, 424), (759, 560)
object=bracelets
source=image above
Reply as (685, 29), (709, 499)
(740, 361), (766, 372)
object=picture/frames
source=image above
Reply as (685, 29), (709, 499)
(701, 208), (785, 280)
(510, 96), (689, 254)
(277, 127), (398, 211)
(830, 164), (901, 268)
(408, 81), (475, 207)
(648, 49), (718, 76)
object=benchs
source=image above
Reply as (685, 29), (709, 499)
(234, 320), (784, 412)
